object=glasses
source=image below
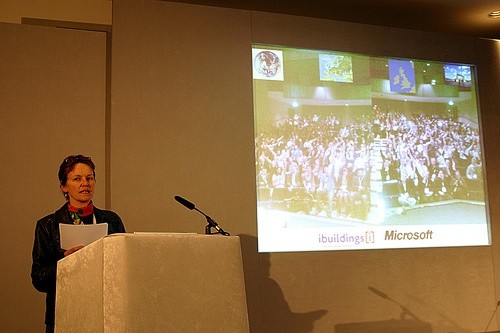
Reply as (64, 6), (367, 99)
(62, 155), (91, 163)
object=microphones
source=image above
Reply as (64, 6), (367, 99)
(174, 195), (225, 235)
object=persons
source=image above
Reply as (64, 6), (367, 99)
(30, 154), (126, 333)
(255, 105), (484, 218)
